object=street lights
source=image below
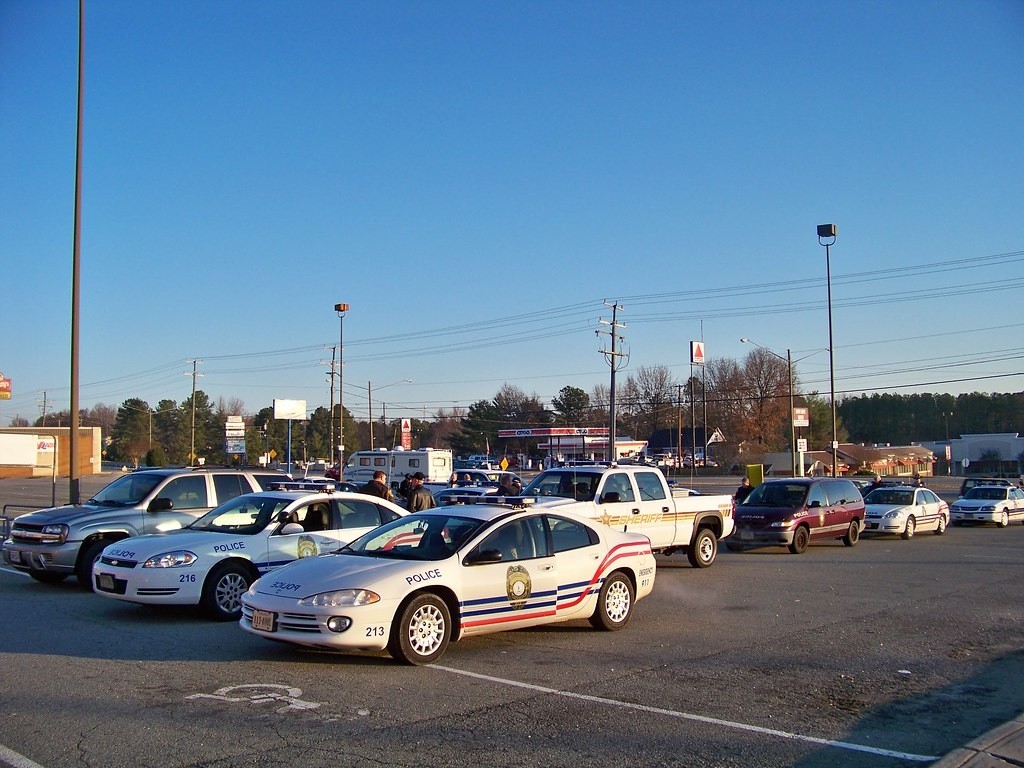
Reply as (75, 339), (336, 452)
(816, 223), (838, 478)
(942, 411), (954, 476)
(741, 339), (830, 478)
(334, 304), (349, 483)
(326, 378), (412, 452)
(114, 402), (185, 449)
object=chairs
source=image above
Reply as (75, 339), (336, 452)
(304, 511), (329, 531)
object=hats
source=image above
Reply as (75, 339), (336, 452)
(411, 472), (425, 480)
(913, 474), (921, 479)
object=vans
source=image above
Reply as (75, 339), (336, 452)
(959, 479), (1013, 499)
(724, 477), (867, 554)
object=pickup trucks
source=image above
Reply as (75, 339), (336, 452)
(519, 460), (736, 568)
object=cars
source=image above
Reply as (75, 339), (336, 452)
(949, 485), (1024, 527)
(239, 495), (657, 666)
(91, 482), (411, 622)
(294, 475), (529, 511)
(851, 480), (950, 541)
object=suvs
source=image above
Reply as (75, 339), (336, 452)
(1, 465), (294, 587)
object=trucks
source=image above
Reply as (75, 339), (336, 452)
(342, 446), (527, 490)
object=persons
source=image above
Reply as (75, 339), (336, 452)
(733, 477), (760, 502)
(358, 471), (395, 503)
(871, 475), (885, 490)
(399, 473), (414, 497)
(464, 474), (470, 480)
(407, 472), (436, 513)
(448, 473), (459, 488)
(495, 475), (513, 496)
(912, 474), (924, 487)
